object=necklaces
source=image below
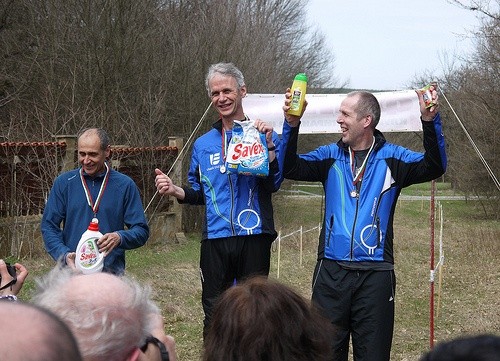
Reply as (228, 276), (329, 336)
(79, 161), (110, 214)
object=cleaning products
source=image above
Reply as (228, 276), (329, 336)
(74, 222), (107, 275)
(225, 120), (269, 178)
(286, 73), (309, 117)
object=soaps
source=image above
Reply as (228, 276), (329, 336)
(418, 84), (435, 110)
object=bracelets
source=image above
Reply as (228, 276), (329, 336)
(0, 295), (17, 301)
(268, 145), (276, 152)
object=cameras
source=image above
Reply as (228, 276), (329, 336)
(0, 262), (16, 284)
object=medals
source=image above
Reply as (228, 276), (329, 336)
(220, 165), (226, 174)
(351, 191), (357, 198)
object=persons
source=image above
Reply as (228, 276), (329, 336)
(28, 266), (179, 361)
(420, 334), (500, 361)
(277, 86), (447, 361)
(41, 126), (149, 277)
(199, 273), (341, 361)
(155, 63), (286, 348)
(0, 301), (83, 361)
(0, 259), (28, 302)
